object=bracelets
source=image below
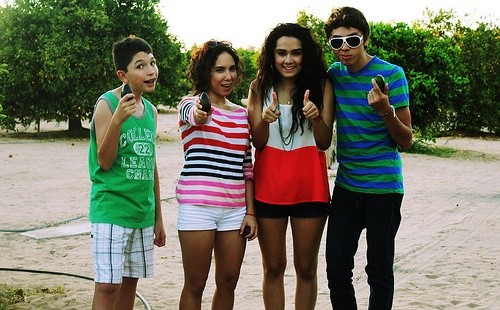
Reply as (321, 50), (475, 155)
(379, 106), (393, 116)
(246, 213), (257, 216)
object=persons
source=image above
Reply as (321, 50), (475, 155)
(246, 22), (336, 310)
(88, 36), (165, 310)
(324, 6), (413, 310)
(175, 39), (259, 310)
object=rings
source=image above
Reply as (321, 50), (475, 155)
(272, 108), (276, 113)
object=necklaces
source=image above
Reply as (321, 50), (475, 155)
(274, 83), (295, 153)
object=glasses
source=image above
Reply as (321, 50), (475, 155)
(208, 41), (232, 50)
(329, 34), (364, 51)
